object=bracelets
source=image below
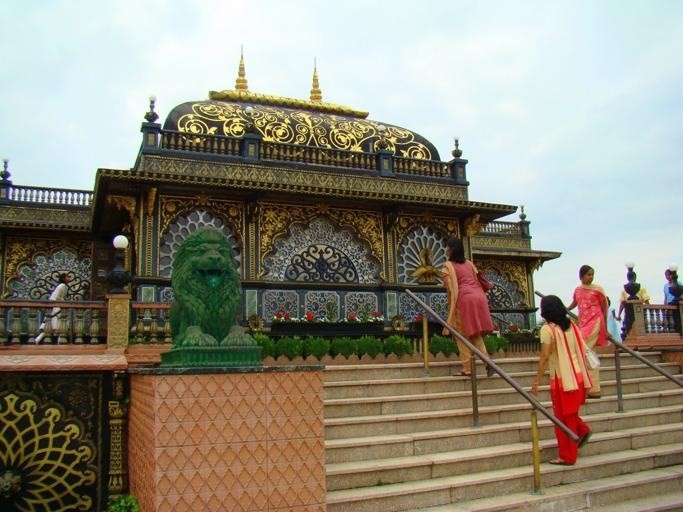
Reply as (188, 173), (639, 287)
(533, 382), (539, 385)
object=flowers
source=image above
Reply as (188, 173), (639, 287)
(414, 310), (446, 321)
(271, 311), (384, 323)
(493, 323), (520, 334)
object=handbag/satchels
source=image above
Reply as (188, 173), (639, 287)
(574, 328), (606, 373)
(470, 263), (495, 290)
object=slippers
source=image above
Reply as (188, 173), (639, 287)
(486, 366), (495, 377)
(585, 392), (601, 400)
(453, 370), (472, 377)
(548, 457), (575, 466)
(578, 428), (593, 449)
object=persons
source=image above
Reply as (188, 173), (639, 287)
(441, 236), (497, 377)
(565, 264), (612, 400)
(33, 272), (71, 346)
(617, 271), (653, 341)
(661, 267), (683, 333)
(530, 294), (593, 466)
(605, 297), (622, 346)
